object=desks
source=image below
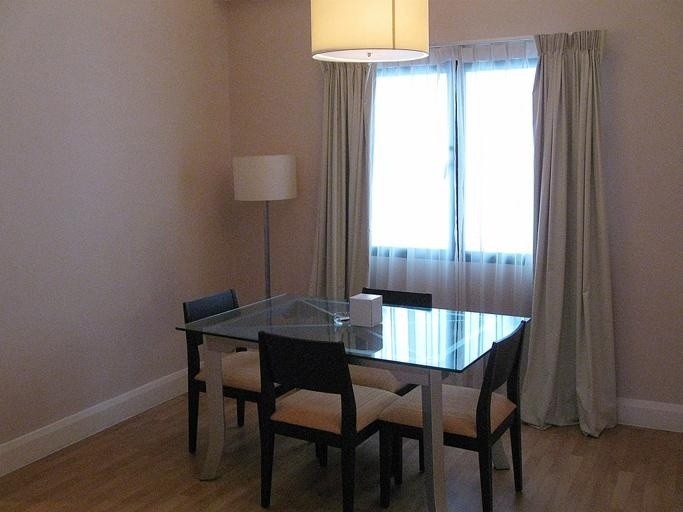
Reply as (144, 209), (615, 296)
(174, 293), (533, 512)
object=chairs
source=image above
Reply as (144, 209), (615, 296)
(183, 289), (299, 463)
(257, 331), (404, 510)
(379, 317), (528, 508)
(351, 287), (433, 474)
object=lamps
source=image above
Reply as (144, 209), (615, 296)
(310, 0), (431, 63)
(232, 153), (301, 295)
